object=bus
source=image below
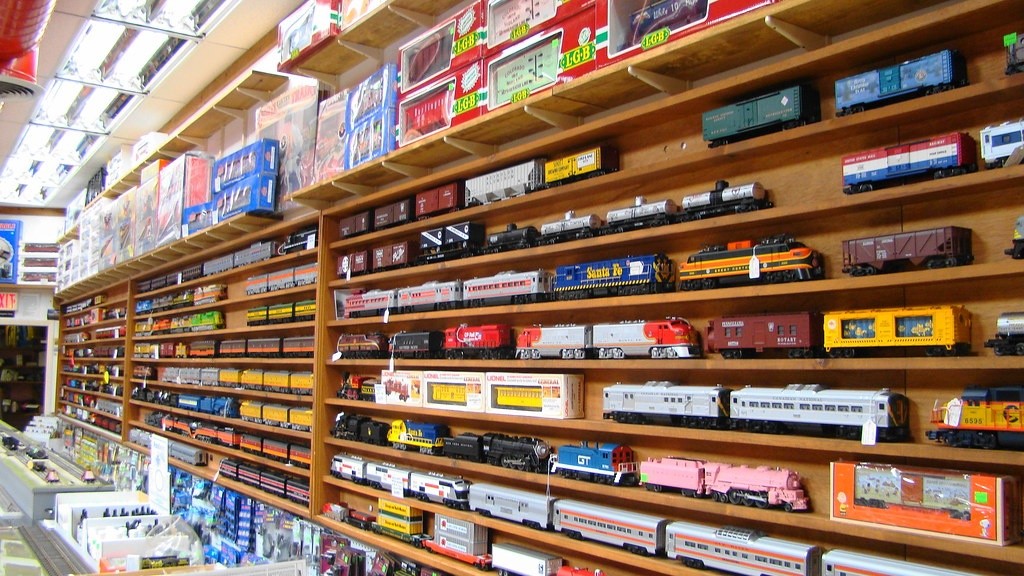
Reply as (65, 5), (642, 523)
(56, 0), (1024, 576)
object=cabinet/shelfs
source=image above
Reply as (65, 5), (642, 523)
(316, 0), (1024, 576)
(55, 277), (130, 444)
(0, 327), (47, 432)
(123, 208), (321, 522)
(284, 0), (945, 211)
(53, 70), (288, 300)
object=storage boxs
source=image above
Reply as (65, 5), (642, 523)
(829, 460), (1021, 547)
(484, 0), (594, 60)
(344, 108), (396, 171)
(212, 139), (279, 195)
(483, 5), (596, 115)
(346, 63), (396, 135)
(314, 86), (349, 182)
(255, 80), (336, 213)
(181, 201), (213, 237)
(485, 372), (585, 420)
(141, 159), (173, 185)
(277, 0), (342, 78)
(212, 173), (276, 224)
(134, 174), (159, 258)
(57, 152), (141, 292)
(382, 369), (423, 409)
(396, 59), (483, 149)
(397, 0), (484, 98)
(423, 370), (485, 413)
(595, 0), (784, 71)
(132, 131), (168, 168)
(154, 153), (215, 249)
